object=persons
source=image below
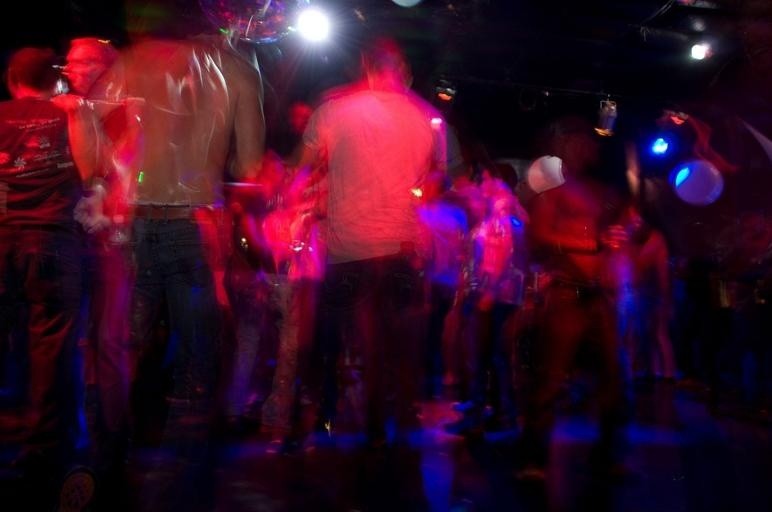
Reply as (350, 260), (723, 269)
(2, 2), (770, 510)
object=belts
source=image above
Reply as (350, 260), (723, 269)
(129, 203), (194, 222)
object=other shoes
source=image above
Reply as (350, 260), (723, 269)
(207, 415), (643, 470)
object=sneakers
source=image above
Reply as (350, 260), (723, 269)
(55, 470), (94, 512)
